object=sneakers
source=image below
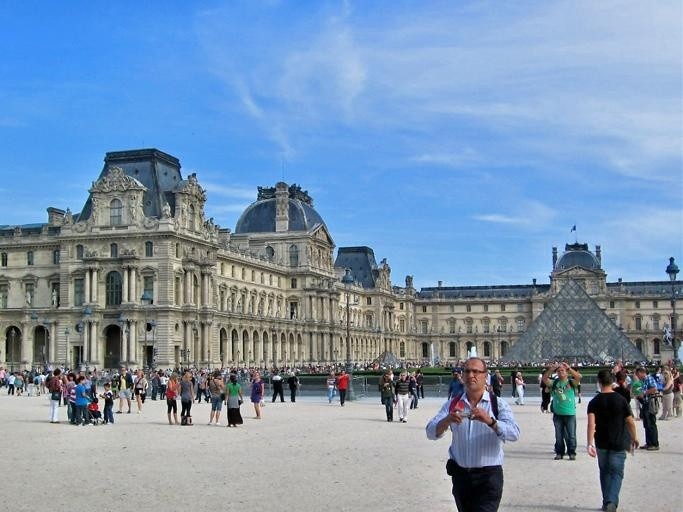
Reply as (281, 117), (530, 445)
(50, 420), (115, 425)
(569, 453), (577, 459)
(606, 501), (617, 512)
(208, 422), (220, 425)
(640, 444), (663, 452)
(554, 451), (564, 461)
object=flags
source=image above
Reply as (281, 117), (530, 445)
(570, 225), (576, 232)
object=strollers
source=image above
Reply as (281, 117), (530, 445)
(83, 398), (106, 425)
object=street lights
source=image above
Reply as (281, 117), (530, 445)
(140, 290), (153, 376)
(339, 266), (355, 401)
(664, 256), (680, 364)
(10, 326), (16, 375)
(204, 351), (382, 369)
(185, 348), (190, 370)
(332, 347), (338, 373)
(63, 325), (70, 369)
(81, 302), (92, 378)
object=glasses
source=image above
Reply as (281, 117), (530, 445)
(463, 369), (488, 375)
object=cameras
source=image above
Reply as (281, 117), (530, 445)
(555, 362), (563, 368)
(456, 411), (471, 419)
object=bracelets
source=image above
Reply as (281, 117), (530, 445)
(486, 417), (496, 427)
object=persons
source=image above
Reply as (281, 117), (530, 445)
(585, 369), (639, 512)
(433, 353), (682, 462)
(424, 357), (522, 512)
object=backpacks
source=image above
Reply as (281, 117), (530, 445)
(34, 378), (39, 385)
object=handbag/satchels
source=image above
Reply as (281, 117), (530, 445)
(180, 414), (196, 426)
(647, 393), (661, 415)
(51, 389), (63, 402)
(221, 392), (226, 400)
